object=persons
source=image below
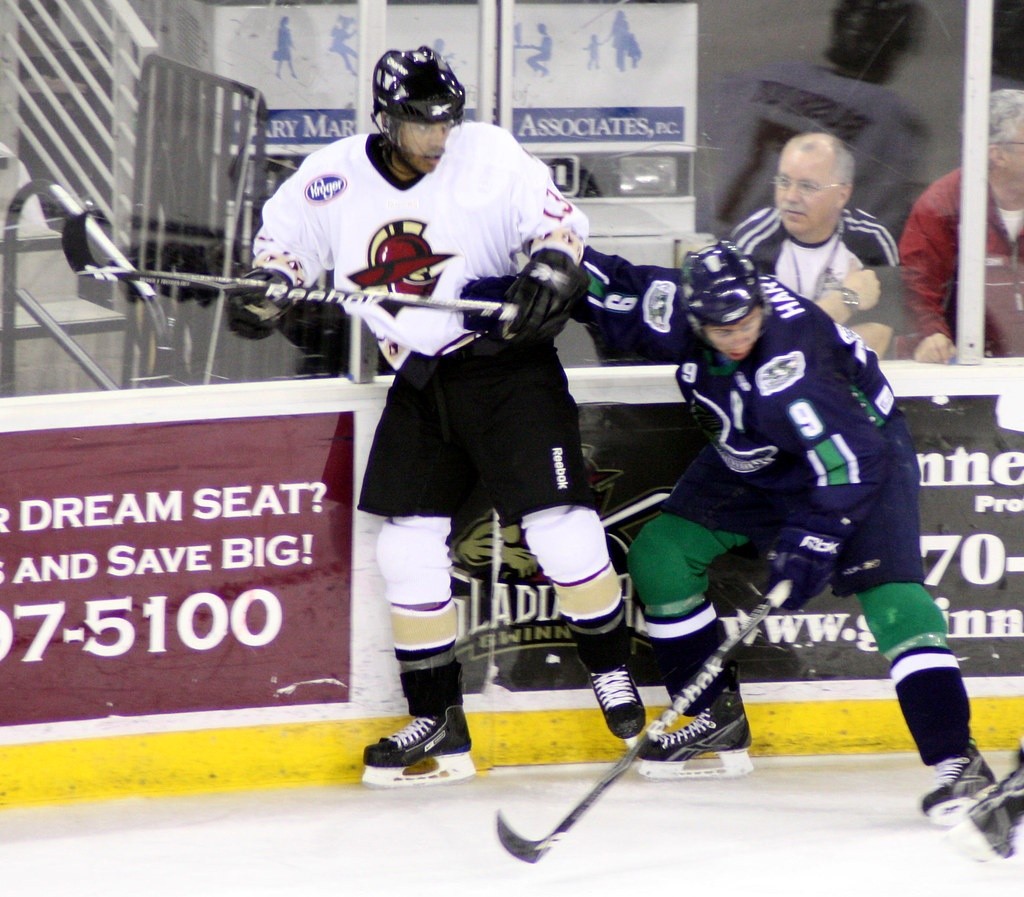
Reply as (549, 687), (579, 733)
(458, 240), (999, 829)
(731, 131), (905, 359)
(224, 45), (646, 789)
(898, 88), (1024, 364)
(715, 0), (927, 241)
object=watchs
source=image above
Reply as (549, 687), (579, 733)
(838, 286), (860, 312)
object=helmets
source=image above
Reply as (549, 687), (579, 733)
(681, 240), (764, 332)
(372, 45), (466, 126)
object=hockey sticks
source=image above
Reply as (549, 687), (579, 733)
(60, 210), (517, 317)
(494, 580), (792, 864)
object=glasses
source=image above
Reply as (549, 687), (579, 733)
(775, 176), (843, 196)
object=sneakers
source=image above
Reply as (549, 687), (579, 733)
(921, 738), (999, 826)
(362, 663), (476, 787)
(635, 659), (754, 779)
(574, 648), (647, 750)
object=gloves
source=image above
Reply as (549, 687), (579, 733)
(226, 269), (292, 340)
(760, 527), (840, 612)
(456, 274), (517, 333)
(499, 249), (592, 344)
